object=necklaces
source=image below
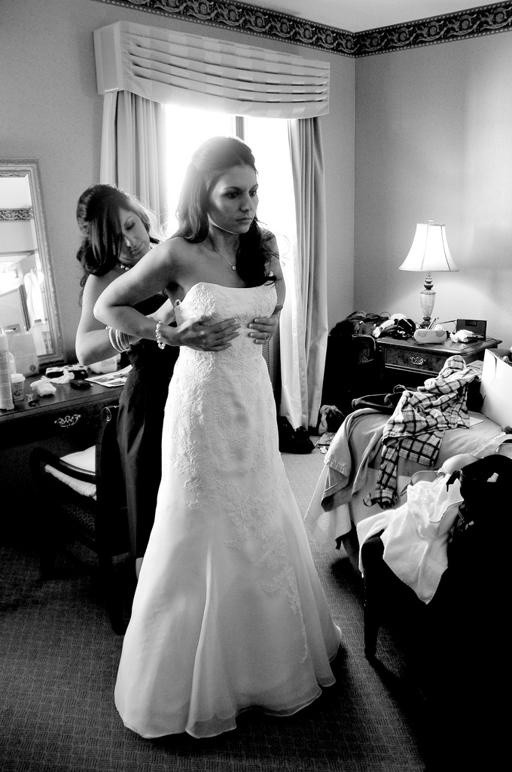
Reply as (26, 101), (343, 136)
(214, 248), (238, 272)
(118, 241), (155, 272)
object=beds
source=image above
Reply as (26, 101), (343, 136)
(307, 406), (512, 581)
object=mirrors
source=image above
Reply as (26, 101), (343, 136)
(0, 158), (66, 364)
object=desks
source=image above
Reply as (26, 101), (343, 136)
(374, 333), (504, 379)
(0, 361), (132, 476)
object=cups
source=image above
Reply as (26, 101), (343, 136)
(11, 374), (25, 401)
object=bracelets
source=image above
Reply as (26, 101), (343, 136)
(154, 319), (166, 352)
(107, 327), (132, 354)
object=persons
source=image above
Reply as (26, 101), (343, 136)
(69, 183), (179, 635)
(92, 136), (349, 746)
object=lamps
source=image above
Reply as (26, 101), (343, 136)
(395, 219), (459, 335)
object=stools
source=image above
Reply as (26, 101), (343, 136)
(360, 522), (499, 668)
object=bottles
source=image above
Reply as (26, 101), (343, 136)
(31, 318), (52, 355)
(0, 336), (15, 409)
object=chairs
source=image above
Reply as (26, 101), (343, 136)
(27, 406), (132, 635)
